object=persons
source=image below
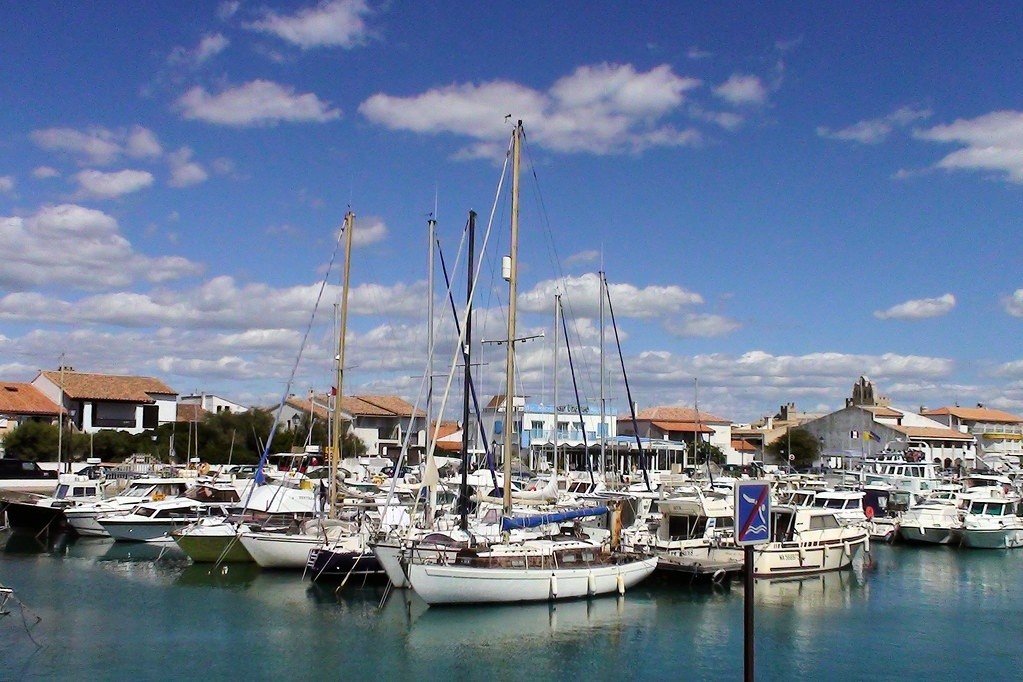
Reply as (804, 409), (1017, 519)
(878, 449), (925, 462)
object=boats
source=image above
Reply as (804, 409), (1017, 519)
(0, 478), (106, 530)
(65, 119), (1023, 605)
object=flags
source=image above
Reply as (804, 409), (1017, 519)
(851, 429), (860, 439)
(864, 430), (881, 443)
